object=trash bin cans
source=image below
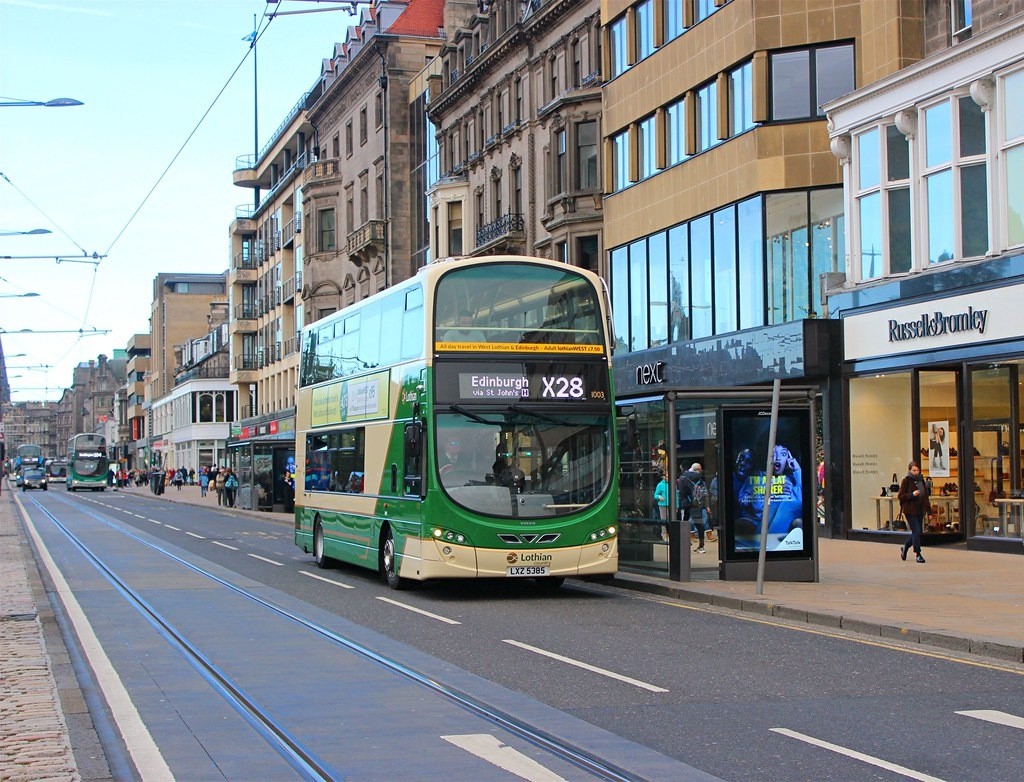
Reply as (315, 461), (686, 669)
(150, 472), (164, 495)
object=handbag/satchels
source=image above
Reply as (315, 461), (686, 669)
(882, 521), (898, 532)
(1001, 441), (1010, 456)
(989, 487), (1006, 502)
(1003, 472), (1010, 479)
(892, 514), (908, 530)
(889, 474), (900, 492)
(1009, 487), (1024, 499)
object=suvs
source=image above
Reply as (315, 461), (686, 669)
(21, 468), (49, 491)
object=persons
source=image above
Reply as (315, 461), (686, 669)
(281, 470), (294, 513)
(442, 310), (487, 342)
(438, 436), (468, 475)
(654, 471), (679, 541)
(200, 464), (233, 507)
(930, 425), (946, 470)
(109, 468), (148, 488)
(735, 425), (803, 536)
(165, 466), (195, 491)
(898, 462), (932, 563)
(678, 460), (718, 554)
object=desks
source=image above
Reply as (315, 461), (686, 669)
(995, 498), (1024, 538)
(870, 495), (955, 531)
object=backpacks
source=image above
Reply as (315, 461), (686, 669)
(683, 474), (708, 509)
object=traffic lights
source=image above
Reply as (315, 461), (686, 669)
(4, 461), (8, 468)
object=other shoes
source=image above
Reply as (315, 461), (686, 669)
(940, 465), (946, 470)
(933, 463), (938, 468)
(900, 546), (908, 560)
(887, 488), (894, 497)
(915, 554), (926, 563)
(922, 445), (983, 533)
(695, 548), (706, 553)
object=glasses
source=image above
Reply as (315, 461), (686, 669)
(449, 440), (462, 446)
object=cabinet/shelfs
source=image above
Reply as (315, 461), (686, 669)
(921, 454), (985, 495)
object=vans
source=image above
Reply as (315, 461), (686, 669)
(44, 456), (67, 483)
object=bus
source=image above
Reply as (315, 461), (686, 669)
(65, 432), (109, 490)
(15, 444), (44, 487)
(294, 253), (640, 592)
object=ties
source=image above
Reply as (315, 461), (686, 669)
(449, 456), (457, 464)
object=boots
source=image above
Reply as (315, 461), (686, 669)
(706, 529), (717, 542)
(880, 487), (887, 496)
(690, 530), (696, 542)
